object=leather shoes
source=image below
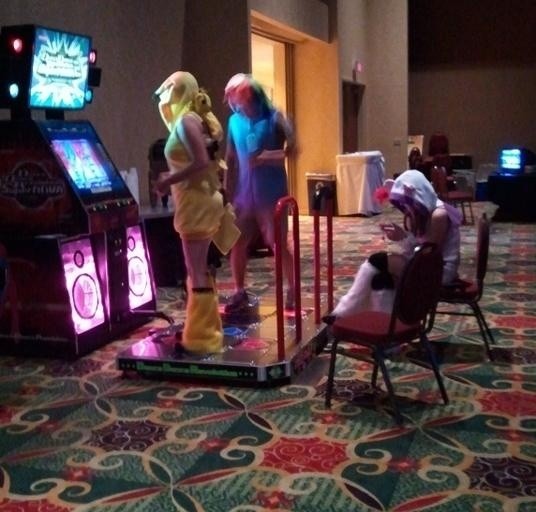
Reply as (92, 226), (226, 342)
(322, 316), (336, 324)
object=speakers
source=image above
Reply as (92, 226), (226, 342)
(3, 233), (107, 340)
(107, 220), (155, 319)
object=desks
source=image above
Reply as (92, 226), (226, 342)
(486, 174), (535, 223)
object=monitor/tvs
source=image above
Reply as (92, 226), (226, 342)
(34, 120), (131, 203)
(497, 144), (527, 174)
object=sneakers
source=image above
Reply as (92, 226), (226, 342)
(285, 289), (295, 309)
(225, 289), (248, 312)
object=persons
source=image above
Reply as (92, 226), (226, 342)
(223, 73), (295, 313)
(322, 169), (463, 359)
(152, 71), (224, 355)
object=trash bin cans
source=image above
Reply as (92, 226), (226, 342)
(305, 172), (336, 216)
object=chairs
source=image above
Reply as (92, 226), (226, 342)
(427, 214), (499, 355)
(317, 244), (449, 426)
(429, 163), (474, 224)
